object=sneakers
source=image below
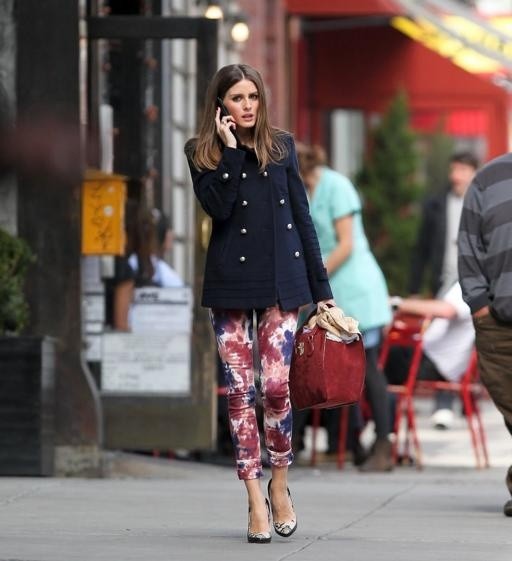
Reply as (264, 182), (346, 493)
(431, 409), (479, 430)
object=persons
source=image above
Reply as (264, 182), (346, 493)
(451, 154), (511, 521)
(382, 278), (476, 388)
(111, 204), (174, 331)
(405, 151), (484, 433)
(183, 62), (340, 548)
(289, 136), (398, 475)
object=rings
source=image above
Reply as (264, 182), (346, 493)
(221, 119), (227, 126)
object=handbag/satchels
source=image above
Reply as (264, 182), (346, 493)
(288, 302), (366, 413)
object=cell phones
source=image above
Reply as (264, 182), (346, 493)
(216, 96), (236, 135)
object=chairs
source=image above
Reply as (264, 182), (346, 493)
(402, 343), (490, 470)
(309, 313), (423, 468)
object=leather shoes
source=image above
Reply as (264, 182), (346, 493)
(358, 442), (393, 471)
(268, 478), (297, 537)
(315, 443), (365, 466)
(247, 498), (271, 543)
